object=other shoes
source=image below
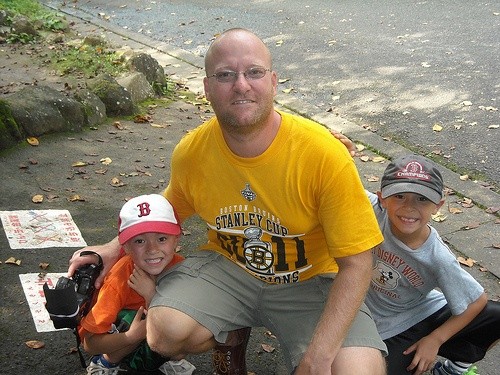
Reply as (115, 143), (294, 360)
(430, 360), (480, 375)
(212, 326), (252, 375)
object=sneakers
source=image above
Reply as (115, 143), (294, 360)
(85, 359), (120, 375)
(159, 359), (197, 375)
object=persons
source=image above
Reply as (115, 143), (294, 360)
(330, 131), (500, 375)
(67, 29), (388, 375)
(77, 193), (196, 375)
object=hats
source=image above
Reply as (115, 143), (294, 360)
(381, 155), (443, 205)
(118, 194), (182, 245)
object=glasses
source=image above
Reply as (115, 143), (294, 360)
(206, 66), (271, 83)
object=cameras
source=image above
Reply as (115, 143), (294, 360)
(53, 264), (99, 327)
